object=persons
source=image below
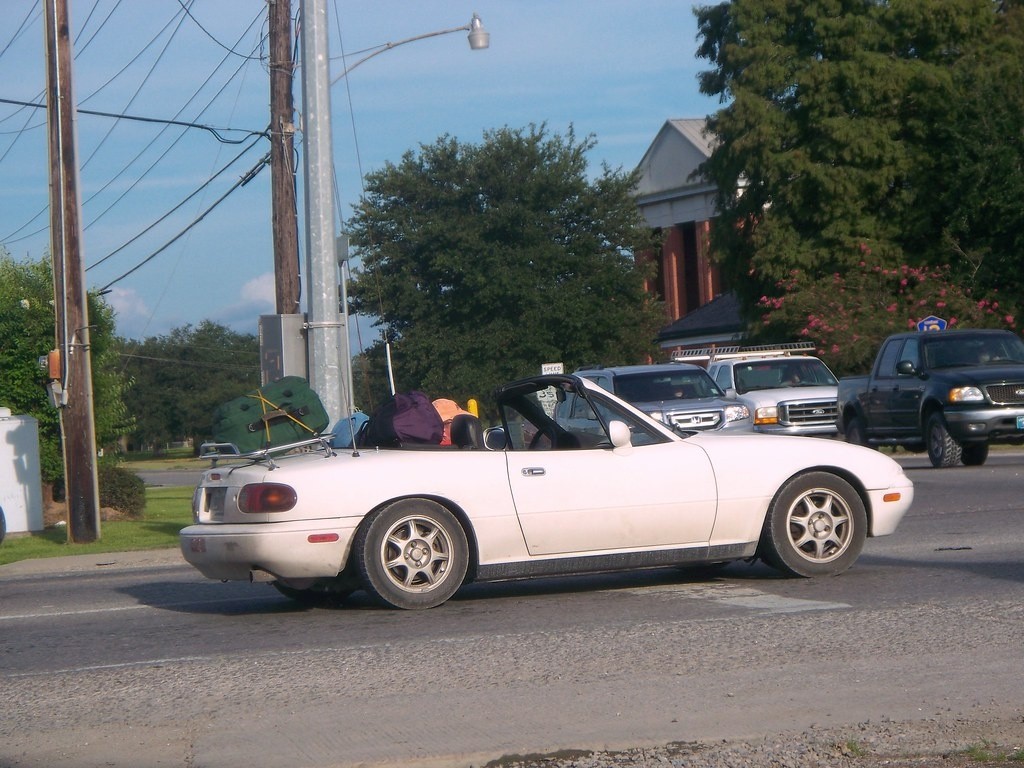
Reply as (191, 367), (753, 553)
(977, 347), (1000, 362)
(672, 385), (685, 399)
(791, 367), (807, 384)
(432, 399), (474, 445)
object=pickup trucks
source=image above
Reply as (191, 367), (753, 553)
(833, 328), (1024, 467)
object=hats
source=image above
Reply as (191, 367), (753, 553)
(433, 399), (475, 422)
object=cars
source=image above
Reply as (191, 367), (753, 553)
(698, 353), (845, 439)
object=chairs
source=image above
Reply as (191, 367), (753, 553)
(451, 414), (485, 450)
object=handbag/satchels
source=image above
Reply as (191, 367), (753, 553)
(333, 405), (370, 448)
(374, 391), (444, 445)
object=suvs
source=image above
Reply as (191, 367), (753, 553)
(548, 363), (757, 448)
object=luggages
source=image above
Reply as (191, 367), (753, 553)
(212, 376), (330, 455)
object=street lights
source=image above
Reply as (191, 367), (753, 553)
(286, 11), (488, 445)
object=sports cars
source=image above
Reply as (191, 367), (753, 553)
(176, 371), (920, 612)
